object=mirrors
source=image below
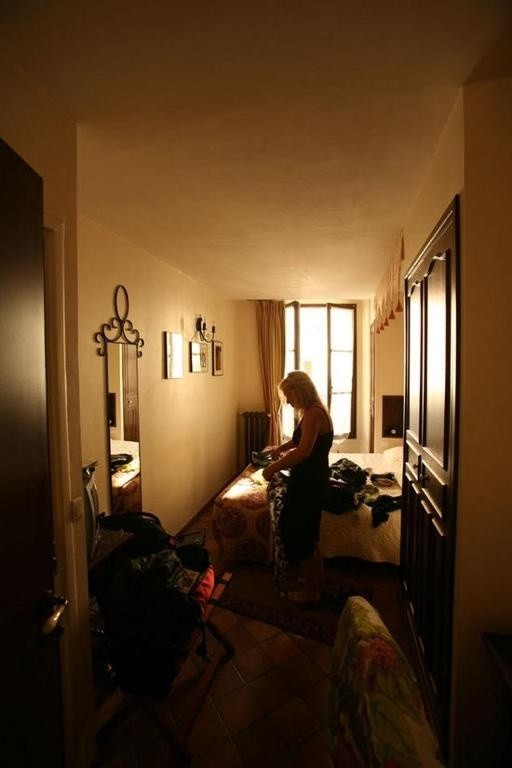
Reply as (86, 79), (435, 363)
(92, 282), (144, 515)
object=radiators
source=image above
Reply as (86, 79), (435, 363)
(237, 411), (272, 466)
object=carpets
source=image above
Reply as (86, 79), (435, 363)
(177, 502), (418, 647)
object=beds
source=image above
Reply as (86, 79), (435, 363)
(210, 445), (404, 574)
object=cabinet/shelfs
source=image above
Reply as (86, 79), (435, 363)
(398, 192), (511, 768)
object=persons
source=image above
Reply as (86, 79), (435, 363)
(260, 369), (334, 606)
